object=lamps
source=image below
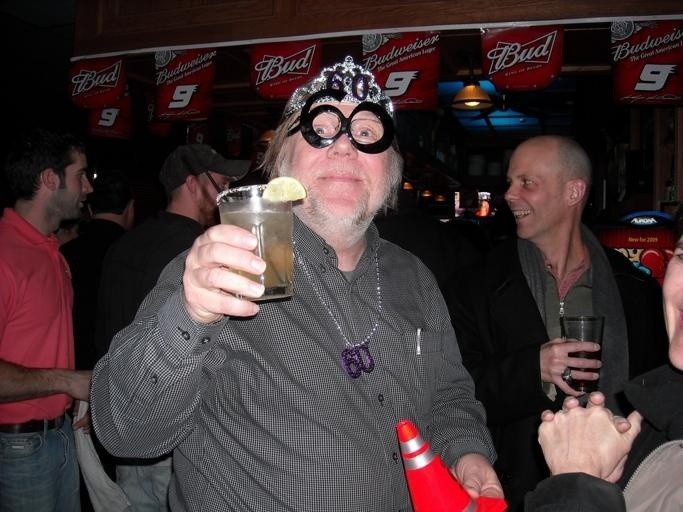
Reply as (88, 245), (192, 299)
(448, 66), (496, 114)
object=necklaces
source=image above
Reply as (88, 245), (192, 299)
(292, 240), (384, 381)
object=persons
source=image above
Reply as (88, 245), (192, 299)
(56, 217), (81, 245)
(59, 166), (146, 286)
(523, 204), (683, 510)
(88, 55), (507, 512)
(0, 131), (93, 512)
(445, 134), (668, 510)
(100, 143), (252, 512)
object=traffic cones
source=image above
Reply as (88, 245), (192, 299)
(395, 419), (508, 511)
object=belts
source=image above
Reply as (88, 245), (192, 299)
(0, 413), (65, 433)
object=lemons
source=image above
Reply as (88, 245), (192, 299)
(262, 176), (307, 204)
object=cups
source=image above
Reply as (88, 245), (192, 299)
(216, 182), (296, 303)
(560, 315), (607, 396)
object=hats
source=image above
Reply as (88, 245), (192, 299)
(158, 143), (252, 193)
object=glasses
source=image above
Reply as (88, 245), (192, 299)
(284, 101), (395, 154)
